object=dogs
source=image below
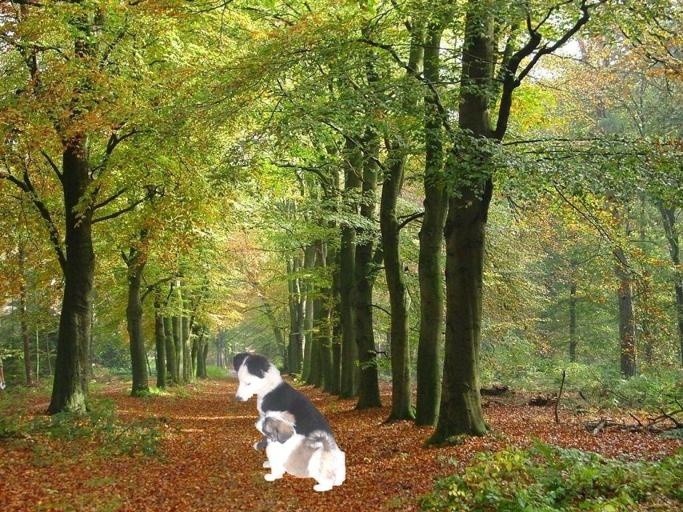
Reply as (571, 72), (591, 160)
(254, 409), (348, 492)
(233, 351), (332, 453)
